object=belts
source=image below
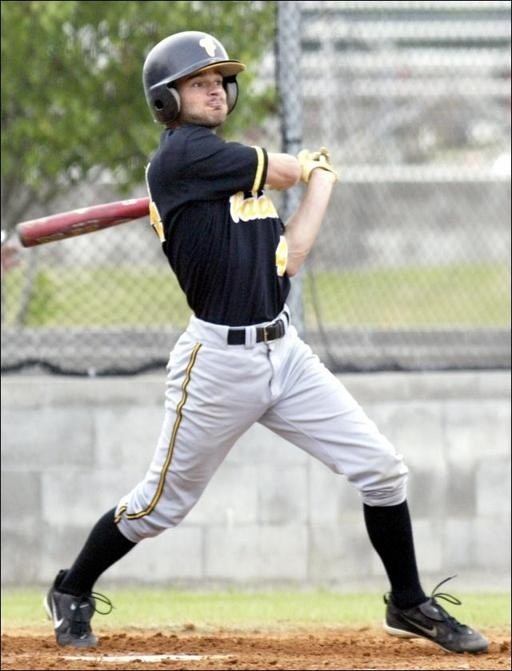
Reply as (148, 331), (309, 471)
(227, 311), (290, 345)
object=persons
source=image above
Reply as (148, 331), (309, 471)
(44, 31), (490, 654)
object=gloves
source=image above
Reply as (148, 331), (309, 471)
(297, 147), (336, 183)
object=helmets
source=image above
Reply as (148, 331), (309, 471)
(142, 30), (247, 124)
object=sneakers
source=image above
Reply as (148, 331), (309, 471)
(383, 594), (489, 654)
(44, 569), (97, 648)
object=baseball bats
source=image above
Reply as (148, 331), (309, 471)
(16, 155), (328, 248)
